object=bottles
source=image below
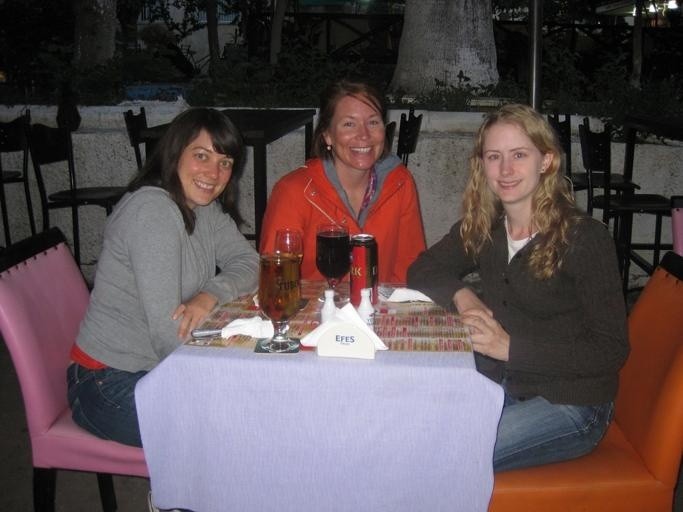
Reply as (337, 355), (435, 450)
(320, 288), (337, 330)
(357, 289), (376, 327)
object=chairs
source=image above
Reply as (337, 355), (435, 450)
(0, 109), (38, 248)
(547, 107), (640, 261)
(396, 106), (424, 168)
(0, 226), (150, 512)
(384, 121), (397, 154)
(122, 106), (148, 170)
(490, 252), (682, 512)
(21, 121), (128, 264)
(579, 116), (674, 295)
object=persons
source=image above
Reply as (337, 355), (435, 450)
(259, 72), (429, 294)
(63, 106), (264, 449)
(404, 101), (632, 476)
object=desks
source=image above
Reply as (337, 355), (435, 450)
(616, 115), (683, 275)
(139, 108), (317, 253)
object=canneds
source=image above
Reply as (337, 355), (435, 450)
(348, 233), (378, 310)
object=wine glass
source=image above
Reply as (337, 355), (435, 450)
(313, 224), (348, 304)
(255, 223), (303, 353)
